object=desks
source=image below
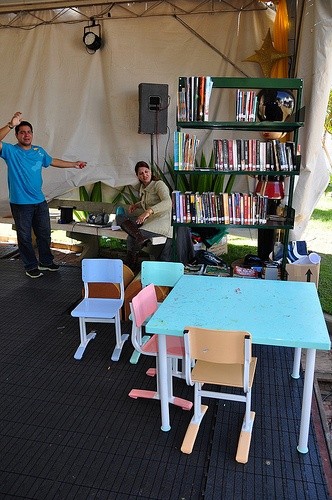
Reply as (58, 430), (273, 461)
(145, 284), (332, 454)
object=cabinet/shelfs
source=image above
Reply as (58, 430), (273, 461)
(176, 77), (305, 280)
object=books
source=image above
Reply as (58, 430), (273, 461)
(236, 89), (258, 122)
(213, 139), (296, 172)
(184, 264), (230, 277)
(172, 190), (268, 225)
(178, 76), (213, 121)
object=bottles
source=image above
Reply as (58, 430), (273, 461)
(117, 207), (125, 226)
(273, 242), (281, 252)
(298, 253), (320, 265)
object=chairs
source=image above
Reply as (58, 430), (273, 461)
(71, 258), (258, 462)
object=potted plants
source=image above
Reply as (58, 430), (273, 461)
(152, 145), (240, 257)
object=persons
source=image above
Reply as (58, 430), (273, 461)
(0, 112), (87, 278)
(116, 161), (172, 274)
(173, 132), (200, 170)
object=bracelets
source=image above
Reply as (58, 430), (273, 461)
(8, 122), (14, 129)
(145, 210), (151, 215)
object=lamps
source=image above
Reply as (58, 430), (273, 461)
(83, 17), (103, 53)
(257, 181), (283, 199)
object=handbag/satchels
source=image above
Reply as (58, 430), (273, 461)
(195, 249), (223, 266)
(288, 241), (308, 264)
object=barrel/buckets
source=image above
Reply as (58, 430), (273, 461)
(57, 205), (76, 220)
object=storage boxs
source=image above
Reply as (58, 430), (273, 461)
(233, 254), (321, 290)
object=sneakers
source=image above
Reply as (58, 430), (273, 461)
(26, 270), (43, 279)
(37, 263), (61, 272)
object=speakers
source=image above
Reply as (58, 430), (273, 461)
(138, 82), (168, 135)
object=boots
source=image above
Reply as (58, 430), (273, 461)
(125, 250), (138, 271)
(120, 219), (152, 253)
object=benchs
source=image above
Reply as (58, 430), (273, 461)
(0, 200), (172, 246)
